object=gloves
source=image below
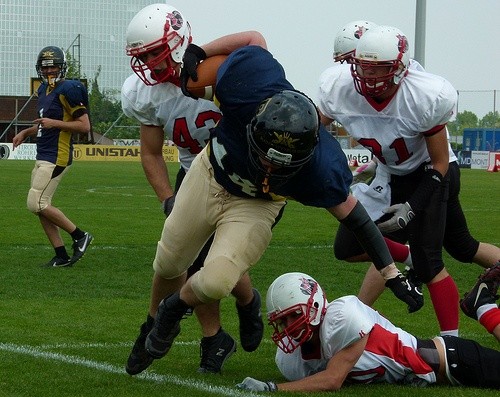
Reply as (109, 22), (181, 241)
(376, 202), (417, 234)
(384, 273), (424, 313)
(235, 376), (277, 394)
(180, 44), (207, 100)
(352, 160), (378, 183)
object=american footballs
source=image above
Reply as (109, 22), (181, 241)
(185, 55), (230, 101)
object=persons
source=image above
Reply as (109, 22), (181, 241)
(121, 4), (265, 374)
(13, 47), (94, 268)
(235, 258), (500, 391)
(316, 27), (462, 338)
(332, 21), (500, 306)
(126, 30), (425, 375)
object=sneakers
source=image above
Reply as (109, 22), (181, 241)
(39, 255), (72, 267)
(70, 231), (94, 264)
(458, 261), (500, 321)
(199, 328), (237, 376)
(401, 265), (423, 293)
(126, 321), (155, 375)
(144, 290), (189, 358)
(235, 287), (264, 352)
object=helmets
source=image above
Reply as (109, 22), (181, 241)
(246, 88), (321, 180)
(265, 272), (328, 354)
(124, 3), (192, 86)
(35, 46), (67, 87)
(333, 20), (377, 65)
(349, 26), (410, 100)
